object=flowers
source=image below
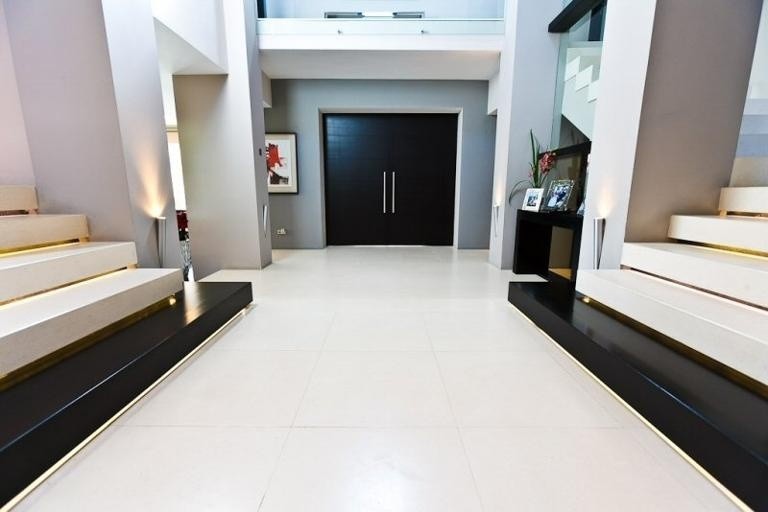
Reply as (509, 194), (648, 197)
(505, 127), (561, 207)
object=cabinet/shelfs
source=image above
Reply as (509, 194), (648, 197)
(511, 207), (583, 301)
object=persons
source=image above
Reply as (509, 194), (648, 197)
(548, 186), (559, 207)
(531, 195), (538, 205)
(555, 186), (565, 205)
(529, 196), (535, 203)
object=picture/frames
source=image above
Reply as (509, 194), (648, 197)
(541, 177), (576, 215)
(518, 186), (545, 214)
(261, 131), (298, 197)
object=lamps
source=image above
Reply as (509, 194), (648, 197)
(592, 215), (606, 273)
(154, 216), (168, 268)
(262, 202), (270, 242)
(492, 203), (499, 239)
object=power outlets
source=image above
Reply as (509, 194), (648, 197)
(275, 229), (287, 236)
